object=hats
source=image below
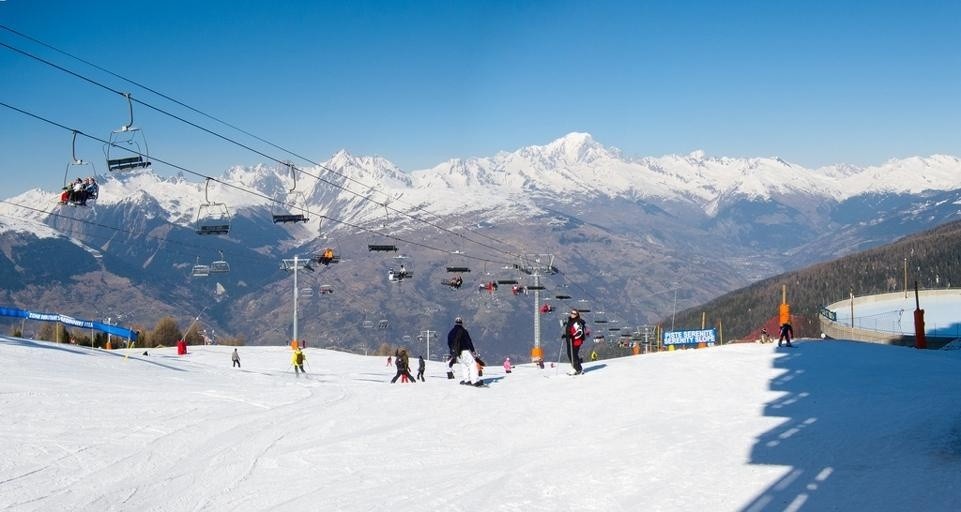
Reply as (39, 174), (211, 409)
(455, 317), (462, 323)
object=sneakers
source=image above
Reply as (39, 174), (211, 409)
(473, 380), (483, 386)
(464, 381), (472, 384)
(575, 369), (582, 374)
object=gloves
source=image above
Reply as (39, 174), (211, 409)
(567, 334), (573, 338)
(562, 334), (567, 338)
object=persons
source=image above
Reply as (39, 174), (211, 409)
(58, 177), (99, 208)
(291, 347), (307, 374)
(562, 309), (586, 375)
(761, 328), (768, 344)
(778, 320), (794, 347)
(504, 357), (515, 373)
(475, 357), (486, 376)
(591, 349), (598, 360)
(445, 355), (457, 379)
(447, 316), (484, 387)
(402, 367), (412, 383)
(449, 276), (637, 350)
(579, 350), (585, 362)
(537, 358), (545, 369)
(232, 348), (241, 368)
(399, 264), (407, 281)
(391, 349), (417, 383)
(386, 356), (393, 367)
(417, 355), (427, 382)
(321, 247), (333, 266)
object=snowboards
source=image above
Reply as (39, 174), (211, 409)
(460, 381), (488, 388)
